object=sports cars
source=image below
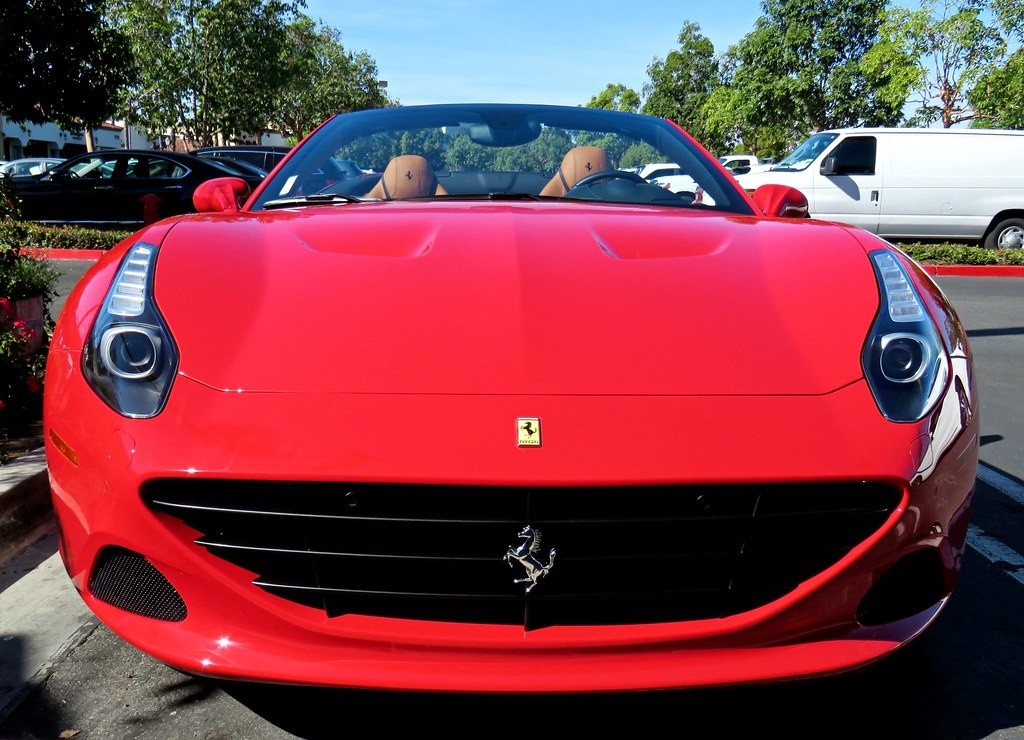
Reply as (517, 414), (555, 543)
(45, 102), (984, 702)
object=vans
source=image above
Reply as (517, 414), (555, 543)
(701, 127), (1024, 249)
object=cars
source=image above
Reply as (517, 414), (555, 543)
(188, 143), (347, 205)
(331, 158), (364, 180)
(635, 154), (814, 204)
(0, 156), (147, 183)
(133, 155), (272, 189)
(12, 148), (272, 230)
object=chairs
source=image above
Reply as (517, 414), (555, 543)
(18, 149), (615, 200)
